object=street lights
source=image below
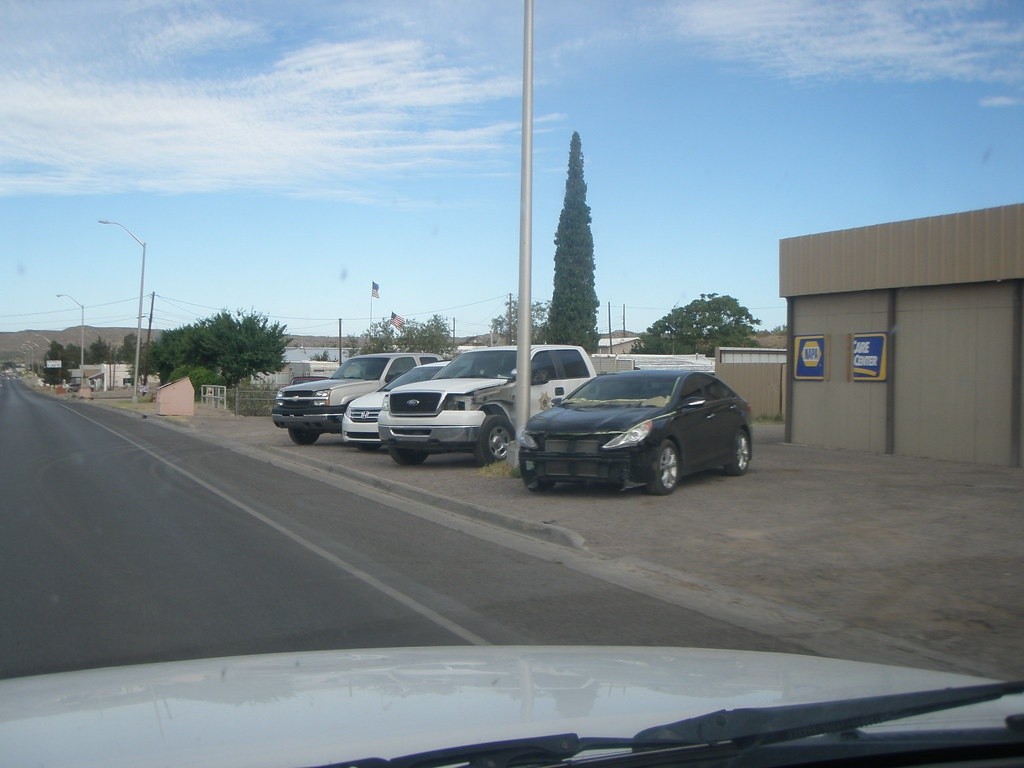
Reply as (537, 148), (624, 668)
(98, 218), (147, 403)
(56, 294), (86, 397)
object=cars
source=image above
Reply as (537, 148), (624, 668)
(518, 368), (756, 495)
(340, 359), (514, 453)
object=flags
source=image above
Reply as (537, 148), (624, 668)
(390, 312), (405, 330)
(372, 281), (379, 298)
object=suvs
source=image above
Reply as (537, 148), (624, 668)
(376, 344), (599, 465)
(269, 351), (445, 446)
